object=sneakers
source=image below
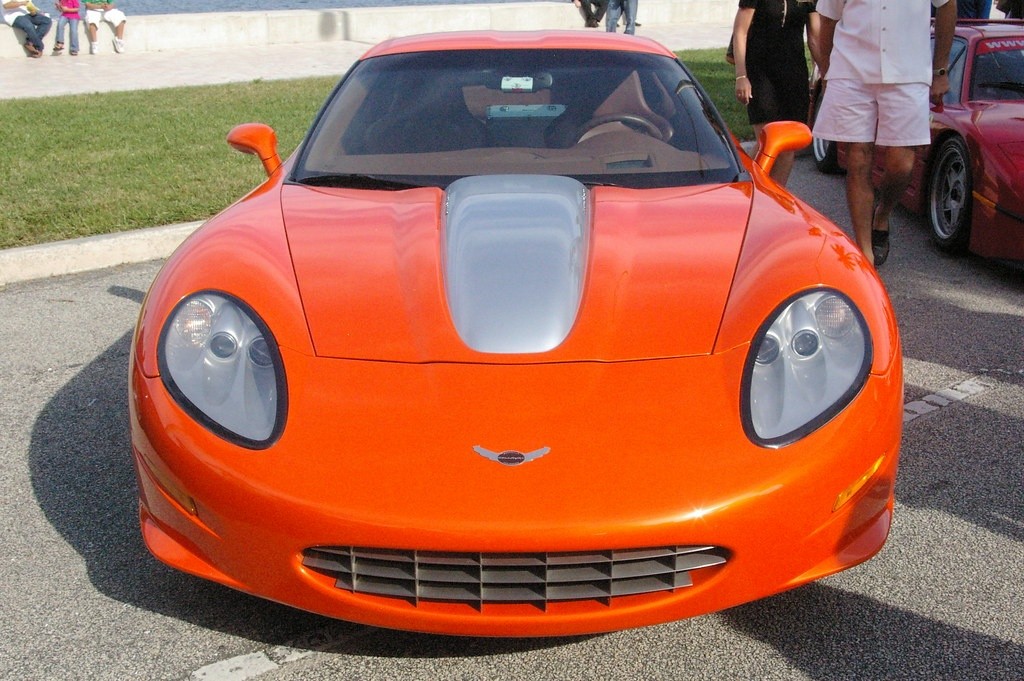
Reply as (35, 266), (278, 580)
(112, 37), (123, 53)
(91, 40), (98, 54)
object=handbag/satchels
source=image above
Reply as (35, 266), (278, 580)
(726, 22), (753, 64)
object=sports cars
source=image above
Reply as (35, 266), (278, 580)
(125, 30), (905, 648)
(801, 17), (1024, 260)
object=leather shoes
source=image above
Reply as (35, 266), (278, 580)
(871, 205), (889, 266)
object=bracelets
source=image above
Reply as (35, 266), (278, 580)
(736, 75), (748, 81)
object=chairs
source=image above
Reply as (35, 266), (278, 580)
(543, 66), (673, 149)
(362, 67), (486, 153)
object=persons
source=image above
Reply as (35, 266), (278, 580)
(0, 0), (53, 59)
(53, 0), (81, 56)
(732, 0), (821, 189)
(81, 0), (127, 54)
(811, 0), (958, 269)
(571, 0), (642, 35)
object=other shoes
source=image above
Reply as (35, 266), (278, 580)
(24, 43), (42, 58)
(588, 17), (598, 27)
(70, 51), (77, 55)
(53, 42), (64, 50)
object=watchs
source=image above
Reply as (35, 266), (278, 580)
(933, 68), (947, 76)
